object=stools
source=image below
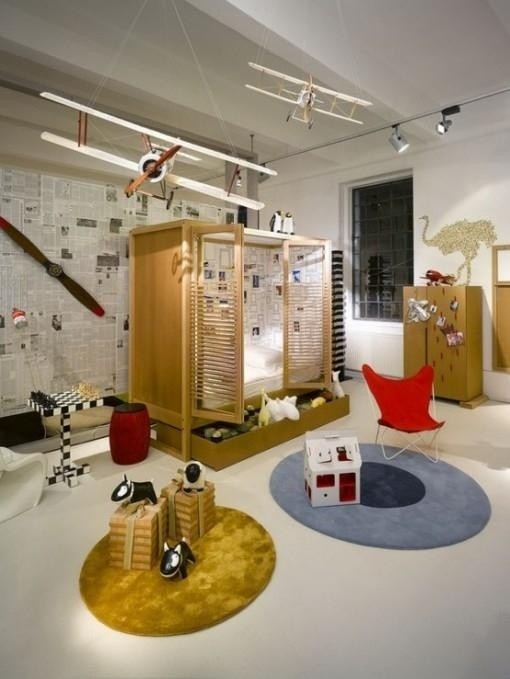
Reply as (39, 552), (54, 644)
(108, 403), (150, 465)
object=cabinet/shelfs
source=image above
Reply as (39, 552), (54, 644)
(398, 278), (481, 407)
(492, 245), (509, 372)
(126, 208), (351, 470)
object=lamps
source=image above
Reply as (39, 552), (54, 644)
(385, 126), (418, 163)
(435, 106), (462, 139)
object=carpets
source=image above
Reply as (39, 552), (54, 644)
(78, 508), (276, 637)
(269, 444), (491, 549)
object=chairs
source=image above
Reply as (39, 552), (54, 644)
(359, 360), (448, 466)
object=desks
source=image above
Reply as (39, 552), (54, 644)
(27, 393), (115, 489)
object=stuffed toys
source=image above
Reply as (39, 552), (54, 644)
(255, 382), (301, 429)
(283, 212), (296, 235)
(106, 474), (161, 510)
(157, 534), (196, 586)
(331, 370), (345, 400)
(176, 458), (207, 498)
(270, 209), (283, 233)
